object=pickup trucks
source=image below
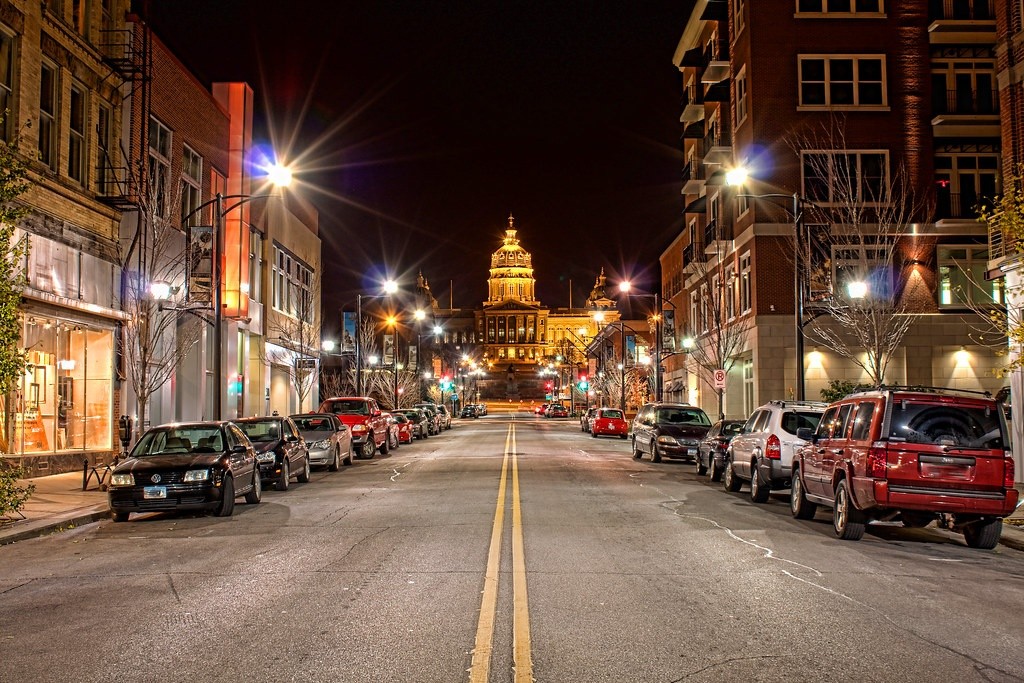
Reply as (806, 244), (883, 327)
(307, 396), (399, 460)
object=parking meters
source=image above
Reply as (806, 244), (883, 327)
(119, 415), (132, 455)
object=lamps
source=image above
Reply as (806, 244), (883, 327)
(813, 346), (819, 354)
(59, 322), (70, 332)
(320, 341), (334, 357)
(959, 345), (967, 354)
(150, 280), (171, 311)
(28, 317), (37, 326)
(45, 319), (51, 329)
(73, 325), (82, 335)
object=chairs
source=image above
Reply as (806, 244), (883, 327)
(181, 438), (192, 452)
(163, 437), (189, 453)
(195, 438), (209, 448)
(670, 413), (695, 422)
(266, 426), (278, 439)
(723, 429), (734, 435)
(407, 413), (418, 421)
(317, 419), (330, 430)
(204, 436), (221, 451)
(396, 417), (404, 423)
(294, 420), (311, 430)
(332, 407), (341, 414)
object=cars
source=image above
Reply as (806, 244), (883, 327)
(286, 413), (353, 473)
(461, 404), (568, 418)
(388, 404), (627, 441)
(211, 415), (310, 491)
(107, 421), (261, 522)
(694, 419), (745, 479)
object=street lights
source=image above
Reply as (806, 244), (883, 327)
(369, 309), (425, 409)
(594, 312), (649, 412)
(323, 280), (397, 397)
(580, 329), (624, 409)
(450, 354), (484, 408)
(621, 280), (693, 402)
(725, 165), (868, 426)
(153, 162), (292, 424)
(398, 324), (442, 402)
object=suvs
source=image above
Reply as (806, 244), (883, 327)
(724, 385), (1019, 551)
(631, 402), (713, 463)
